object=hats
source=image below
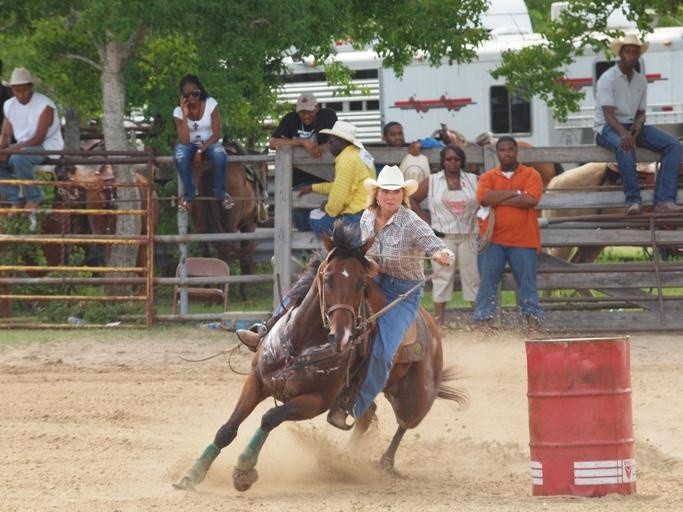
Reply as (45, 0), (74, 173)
(476, 133), (488, 143)
(612, 35), (649, 56)
(319, 121), (363, 148)
(364, 164), (418, 196)
(1, 67), (43, 87)
(296, 93), (317, 112)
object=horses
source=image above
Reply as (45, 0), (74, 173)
(432, 123), (564, 218)
(172, 218), (472, 491)
(188, 142), (269, 308)
(541, 162), (683, 311)
(41, 139), (159, 303)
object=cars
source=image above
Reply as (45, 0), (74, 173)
(89, 106), (154, 138)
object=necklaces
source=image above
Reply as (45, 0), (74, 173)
(185, 101), (202, 131)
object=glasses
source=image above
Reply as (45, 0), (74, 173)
(184, 90), (200, 98)
(446, 157), (460, 161)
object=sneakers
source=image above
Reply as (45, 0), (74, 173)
(177, 197), (189, 211)
(625, 202), (641, 214)
(654, 202), (683, 212)
(327, 408), (346, 427)
(219, 193), (233, 210)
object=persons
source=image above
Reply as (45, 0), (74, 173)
(374, 121), (443, 177)
(234, 165), (455, 428)
(0, 58), (12, 127)
(0, 67), (63, 218)
(268, 92), (337, 273)
(593, 29), (682, 214)
(472, 135), (543, 339)
(171, 74), (234, 212)
(295, 120), (376, 241)
(407, 145), (480, 325)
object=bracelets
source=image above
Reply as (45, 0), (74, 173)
(197, 149), (203, 155)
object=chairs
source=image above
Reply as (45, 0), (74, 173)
(171, 256), (230, 314)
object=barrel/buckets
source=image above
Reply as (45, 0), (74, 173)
(521, 334), (638, 500)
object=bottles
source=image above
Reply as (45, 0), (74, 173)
(67, 317), (87, 326)
(198, 322), (221, 330)
